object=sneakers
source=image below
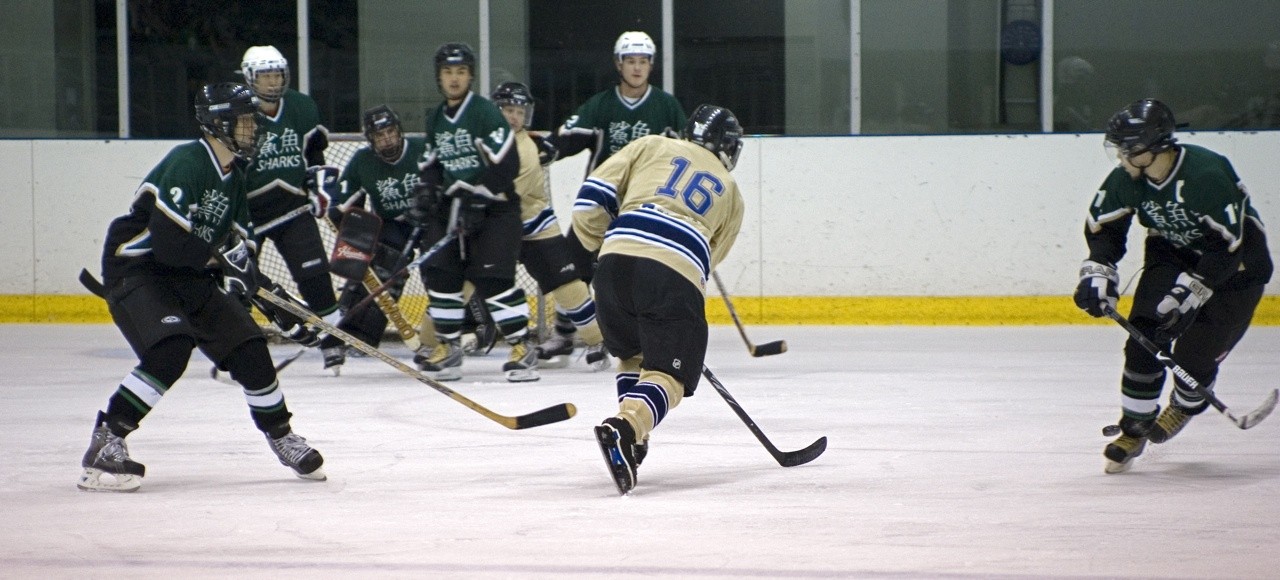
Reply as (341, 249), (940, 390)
(631, 438), (649, 466)
(1146, 404), (1192, 447)
(594, 417), (639, 495)
(534, 325), (576, 369)
(1103, 425), (1150, 474)
(501, 335), (541, 383)
(76, 408), (146, 493)
(320, 339), (345, 377)
(264, 427), (327, 481)
(577, 338), (609, 373)
(417, 335), (465, 379)
(211, 363), (240, 387)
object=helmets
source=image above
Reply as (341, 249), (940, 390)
(195, 81), (257, 157)
(243, 45), (289, 103)
(687, 102), (742, 172)
(362, 106), (404, 159)
(492, 81), (533, 107)
(614, 30), (656, 63)
(434, 43), (478, 100)
(1105, 99), (1175, 160)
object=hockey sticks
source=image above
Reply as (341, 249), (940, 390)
(703, 365), (828, 467)
(712, 270), (788, 358)
(258, 285), (579, 431)
(1099, 300), (1280, 431)
(324, 216), (478, 357)
(77, 202), (313, 300)
(209, 230), (456, 387)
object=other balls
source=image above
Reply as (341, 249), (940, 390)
(1103, 424), (1120, 437)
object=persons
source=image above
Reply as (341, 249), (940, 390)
(1072, 98), (1274, 465)
(238, 46), (346, 368)
(80, 82), (323, 475)
(327, 32), (687, 372)
(572, 104), (746, 495)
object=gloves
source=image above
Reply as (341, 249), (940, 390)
(409, 185), (437, 229)
(1074, 256), (1115, 319)
(532, 136), (553, 169)
(211, 231), (257, 302)
(261, 284), (320, 347)
(453, 194), (488, 237)
(304, 168), (332, 219)
(1154, 271), (1212, 344)
